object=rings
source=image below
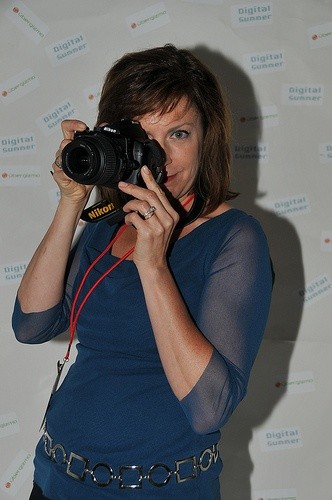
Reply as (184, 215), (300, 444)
(54, 159), (63, 169)
(144, 206), (156, 221)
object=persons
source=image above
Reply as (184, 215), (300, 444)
(12, 46), (275, 500)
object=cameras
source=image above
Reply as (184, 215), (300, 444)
(60, 119), (166, 192)
